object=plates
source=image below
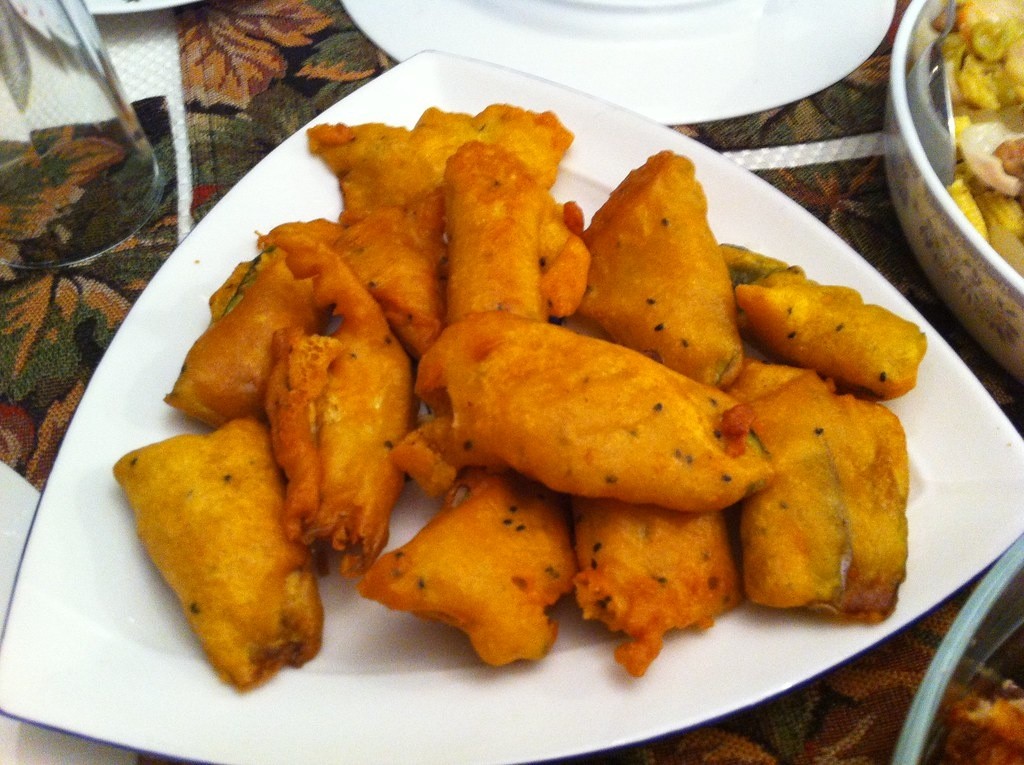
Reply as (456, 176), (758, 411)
(0, 48), (1024, 763)
(1, 464), (145, 765)
(340, 3), (892, 125)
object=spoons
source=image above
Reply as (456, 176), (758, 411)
(904, 2), (958, 186)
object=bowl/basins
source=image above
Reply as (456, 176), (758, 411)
(888, 0), (1022, 379)
(892, 537), (1023, 765)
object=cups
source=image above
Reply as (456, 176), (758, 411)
(3, 0), (163, 267)
